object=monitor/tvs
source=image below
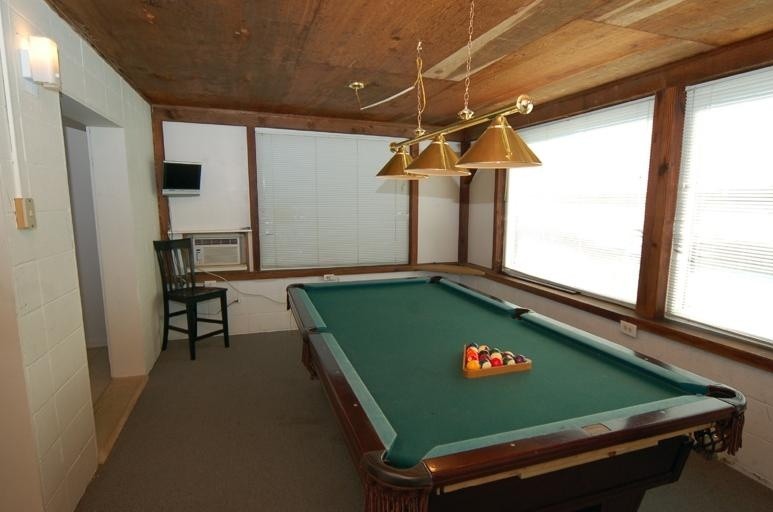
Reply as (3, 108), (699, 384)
(161, 160), (204, 196)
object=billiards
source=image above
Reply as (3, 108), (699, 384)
(467, 342), (525, 370)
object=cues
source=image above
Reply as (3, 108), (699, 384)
(521, 443), (657, 474)
(436, 421), (716, 496)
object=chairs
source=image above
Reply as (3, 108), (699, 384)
(153, 237), (230, 362)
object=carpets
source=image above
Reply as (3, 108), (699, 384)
(70, 329), (771, 510)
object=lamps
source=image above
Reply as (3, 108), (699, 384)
(20, 34), (61, 87)
(374, 93), (543, 180)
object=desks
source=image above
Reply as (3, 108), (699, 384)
(285, 274), (750, 511)
